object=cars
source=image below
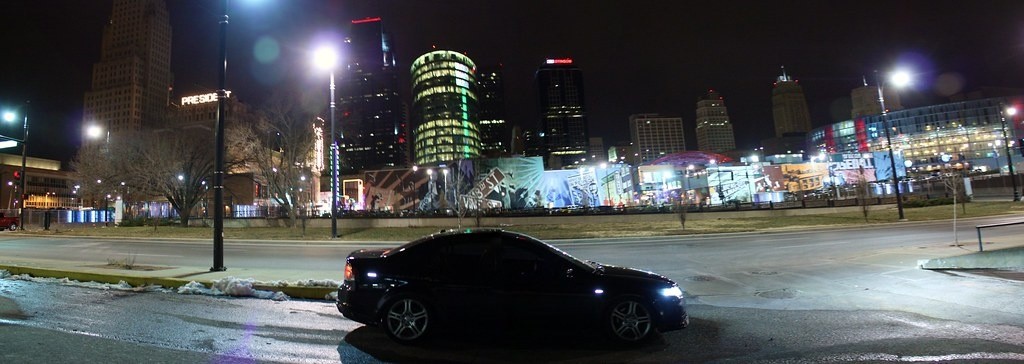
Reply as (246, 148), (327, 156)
(336, 227), (689, 350)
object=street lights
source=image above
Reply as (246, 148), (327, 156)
(4, 99), (31, 231)
(314, 44), (340, 239)
(872, 68), (909, 223)
(87, 125), (109, 227)
(998, 100), (1024, 201)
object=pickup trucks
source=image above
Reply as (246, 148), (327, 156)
(0, 209), (20, 231)
